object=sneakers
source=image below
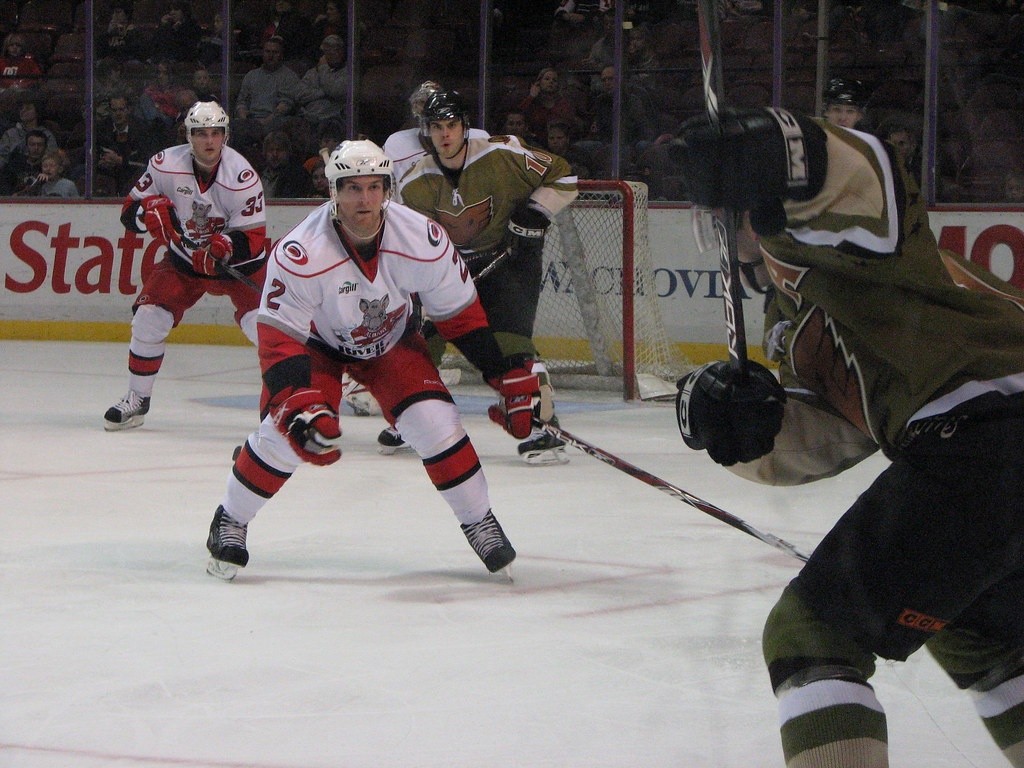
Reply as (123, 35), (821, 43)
(517, 416), (571, 465)
(205, 506), (250, 580)
(104, 392), (151, 431)
(378, 426), (413, 455)
(460, 509), (517, 585)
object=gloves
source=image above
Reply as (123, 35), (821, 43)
(268, 385), (341, 465)
(680, 108), (829, 239)
(484, 353), (543, 438)
(192, 235), (233, 277)
(141, 194), (184, 246)
(676, 359), (787, 465)
(498, 209), (552, 271)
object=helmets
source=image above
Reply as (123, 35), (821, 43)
(419, 92), (467, 120)
(412, 80), (450, 105)
(325, 139), (401, 202)
(820, 76), (866, 116)
(183, 100), (230, 147)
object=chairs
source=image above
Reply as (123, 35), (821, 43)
(0, 0), (1024, 201)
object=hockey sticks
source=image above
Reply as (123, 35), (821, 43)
(527, 413), (810, 567)
(694, 1), (755, 384)
(341, 240), (524, 399)
(181, 228), (262, 292)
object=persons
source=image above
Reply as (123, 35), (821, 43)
(763, 76), (869, 389)
(634, 106), (1024, 768)
(101, 99), (349, 431)
(380, 79), (492, 202)
(0, 0), (1024, 202)
(375, 90), (580, 461)
(202, 139), (540, 583)
